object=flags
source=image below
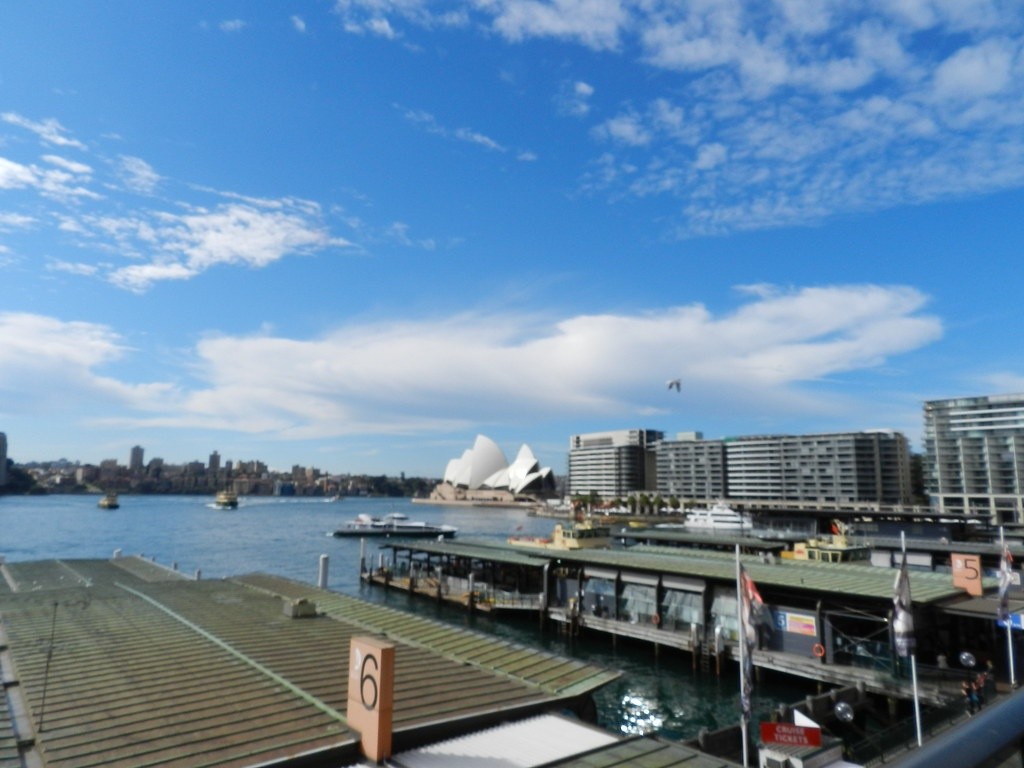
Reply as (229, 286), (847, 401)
(890, 549), (918, 659)
(736, 557), (780, 726)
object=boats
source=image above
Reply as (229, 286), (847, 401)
(330, 502), (458, 539)
(569, 504), (615, 525)
(779, 519), (875, 568)
(506, 519), (613, 552)
(214, 489), (239, 511)
(684, 498), (752, 529)
(98, 492), (120, 510)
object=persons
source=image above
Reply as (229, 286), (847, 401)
(959, 659), (998, 718)
